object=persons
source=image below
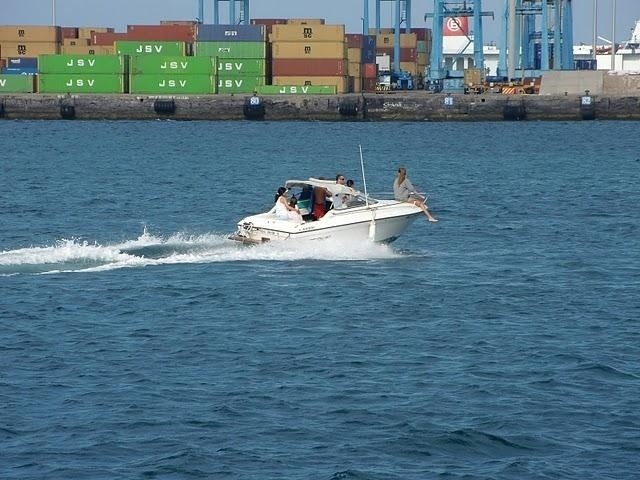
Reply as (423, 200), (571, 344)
(273, 174), (355, 224)
(392, 167), (439, 223)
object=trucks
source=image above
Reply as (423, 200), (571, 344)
(462, 66), (487, 96)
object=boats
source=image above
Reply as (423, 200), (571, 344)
(227, 147), (432, 252)
